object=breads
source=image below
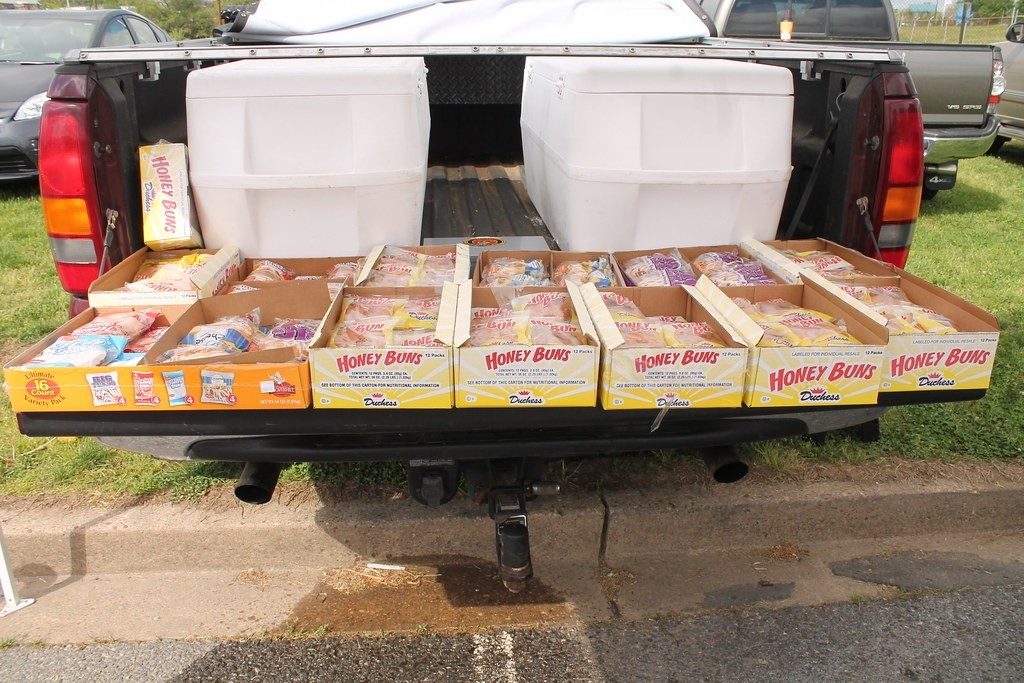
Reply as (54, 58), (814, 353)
(17, 251), (966, 368)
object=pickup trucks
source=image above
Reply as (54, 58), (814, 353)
(10, 0), (988, 602)
(686, 0), (1006, 204)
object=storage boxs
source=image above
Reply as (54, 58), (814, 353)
(516, 55), (792, 251)
(3, 142), (1001, 413)
(183, 55), (428, 261)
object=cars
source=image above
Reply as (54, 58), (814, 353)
(972, 16), (1024, 155)
(0, 9), (182, 186)
